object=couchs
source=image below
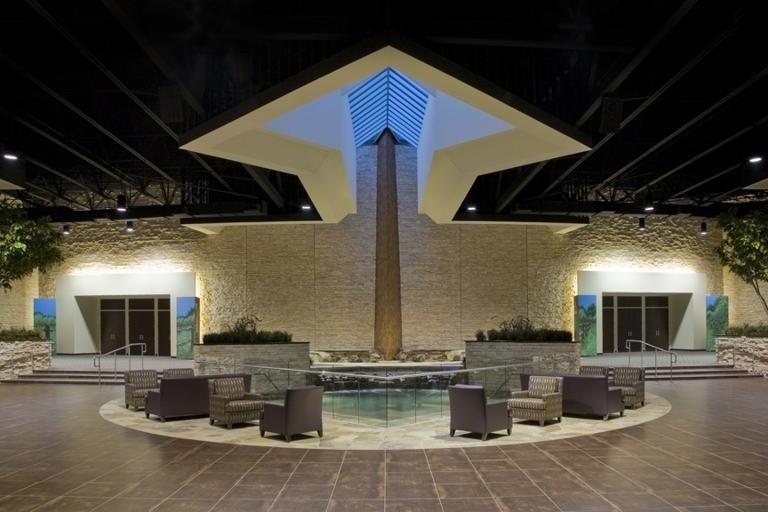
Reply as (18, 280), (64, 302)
(521, 373), (625, 420)
(145, 373), (253, 422)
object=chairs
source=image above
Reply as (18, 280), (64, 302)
(578, 366), (646, 410)
(448, 376), (564, 441)
(122, 367), (194, 410)
(208, 378), (324, 442)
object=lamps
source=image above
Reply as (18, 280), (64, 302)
(637, 184), (707, 236)
(64, 195), (133, 236)
